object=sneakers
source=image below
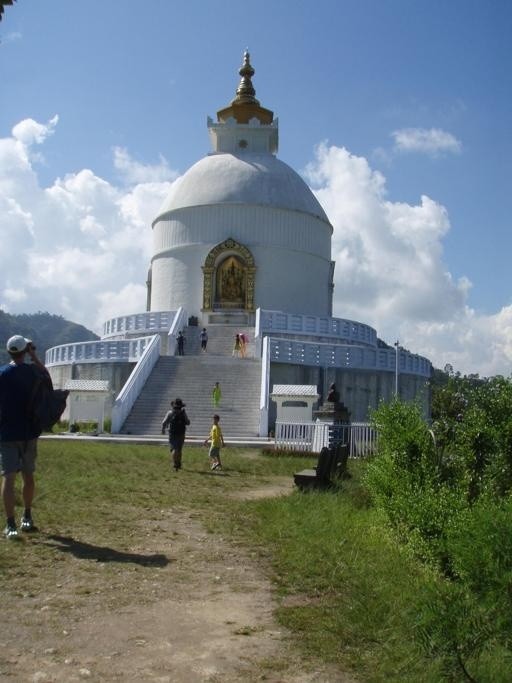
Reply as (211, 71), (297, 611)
(211, 462), (220, 470)
(3, 517), (34, 540)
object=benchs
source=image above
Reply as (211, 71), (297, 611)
(293, 443), (349, 487)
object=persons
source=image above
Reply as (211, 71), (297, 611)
(231, 333), (241, 360)
(1, 334), (53, 537)
(238, 332), (246, 358)
(199, 327), (208, 352)
(176, 330), (187, 355)
(202, 414), (226, 471)
(212, 381), (223, 407)
(161, 397), (191, 471)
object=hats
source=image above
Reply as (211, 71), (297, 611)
(6, 335), (33, 353)
(171, 399), (185, 408)
